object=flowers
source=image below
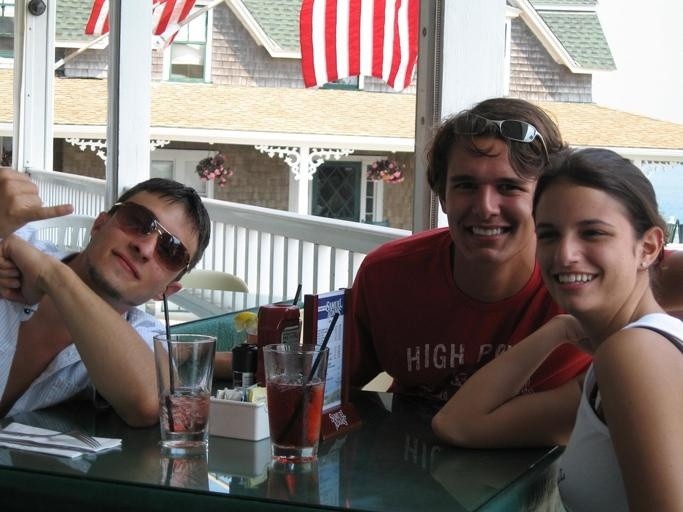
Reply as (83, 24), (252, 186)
(195, 153), (238, 188)
(361, 158), (408, 186)
(232, 310), (259, 348)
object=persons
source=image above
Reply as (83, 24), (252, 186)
(431, 151), (683, 512)
(340, 96), (683, 420)
(0, 167), (211, 428)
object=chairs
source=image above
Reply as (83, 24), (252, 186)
(30, 213), (99, 254)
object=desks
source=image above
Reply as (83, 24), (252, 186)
(0, 382), (569, 512)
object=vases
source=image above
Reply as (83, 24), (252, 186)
(231, 344), (260, 376)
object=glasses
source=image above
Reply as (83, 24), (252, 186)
(447, 109), (552, 162)
(106, 200), (191, 275)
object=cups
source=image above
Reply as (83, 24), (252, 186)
(161, 452), (208, 495)
(263, 343), (330, 462)
(244, 327), (257, 345)
(265, 461), (319, 505)
(155, 330), (218, 447)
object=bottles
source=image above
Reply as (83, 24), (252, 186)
(257, 303), (301, 387)
(230, 342), (255, 387)
